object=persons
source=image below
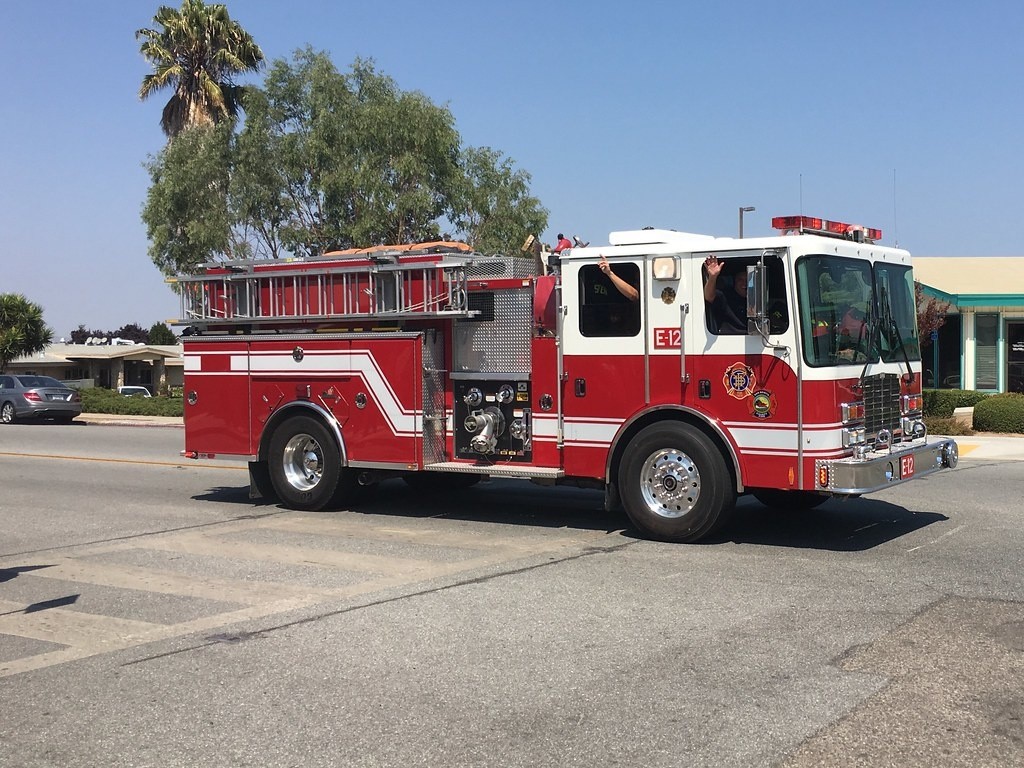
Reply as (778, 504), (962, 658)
(769, 280), (882, 362)
(703, 255), (762, 335)
(581, 255), (640, 336)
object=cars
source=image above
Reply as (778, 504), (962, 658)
(116, 385), (152, 398)
(0, 374), (84, 424)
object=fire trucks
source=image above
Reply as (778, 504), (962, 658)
(162, 214), (961, 545)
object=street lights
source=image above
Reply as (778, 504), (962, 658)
(738, 206), (756, 240)
